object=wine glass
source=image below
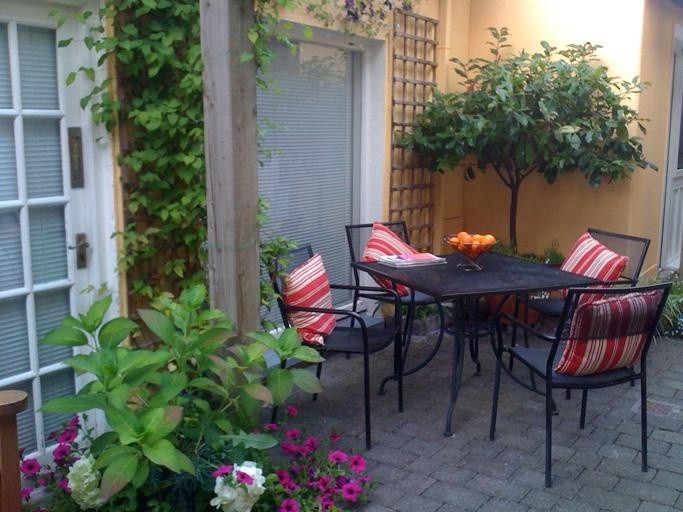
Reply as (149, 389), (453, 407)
(442, 233), (500, 273)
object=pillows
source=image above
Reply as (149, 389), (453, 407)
(281, 251), (337, 346)
(364, 224), (421, 297)
(559, 232), (629, 308)
(554, 290), (662, 376)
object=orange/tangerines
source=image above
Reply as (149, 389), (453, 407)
(450, 231), (496, 257)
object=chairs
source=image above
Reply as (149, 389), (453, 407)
(490, 283), (674, 487)
(345, 221), (462, 382)
(507, 228), (651, 400)
(267, 241), (404, 450)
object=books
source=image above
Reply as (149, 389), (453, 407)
(377, 251), (449, 268)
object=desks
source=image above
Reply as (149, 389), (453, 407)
(351, 252), (606, 436)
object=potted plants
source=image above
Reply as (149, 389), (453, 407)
(389, 25), (658, 327)
